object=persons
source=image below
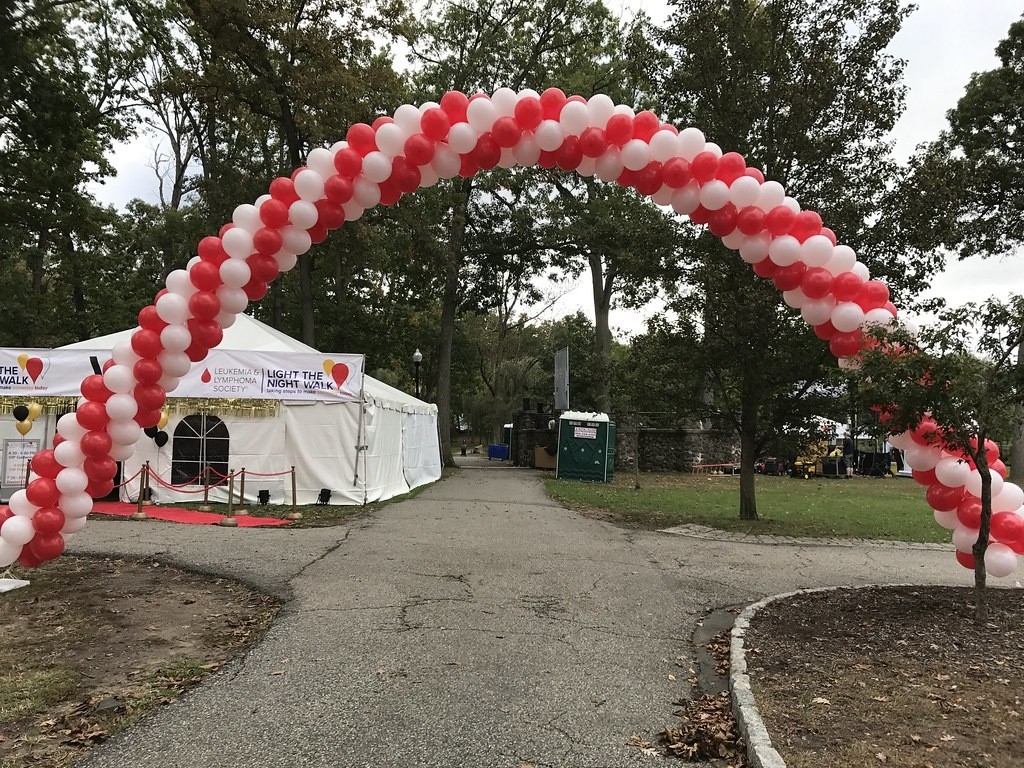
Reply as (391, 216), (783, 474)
(838, 431), (855, 479)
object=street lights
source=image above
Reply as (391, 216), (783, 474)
(413, 347), (424, 398)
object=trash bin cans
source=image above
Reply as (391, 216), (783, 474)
(487, 442), (508, 462)
(534, 428), (558, 469)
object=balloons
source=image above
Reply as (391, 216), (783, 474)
(0, 90), (1024, 577)
